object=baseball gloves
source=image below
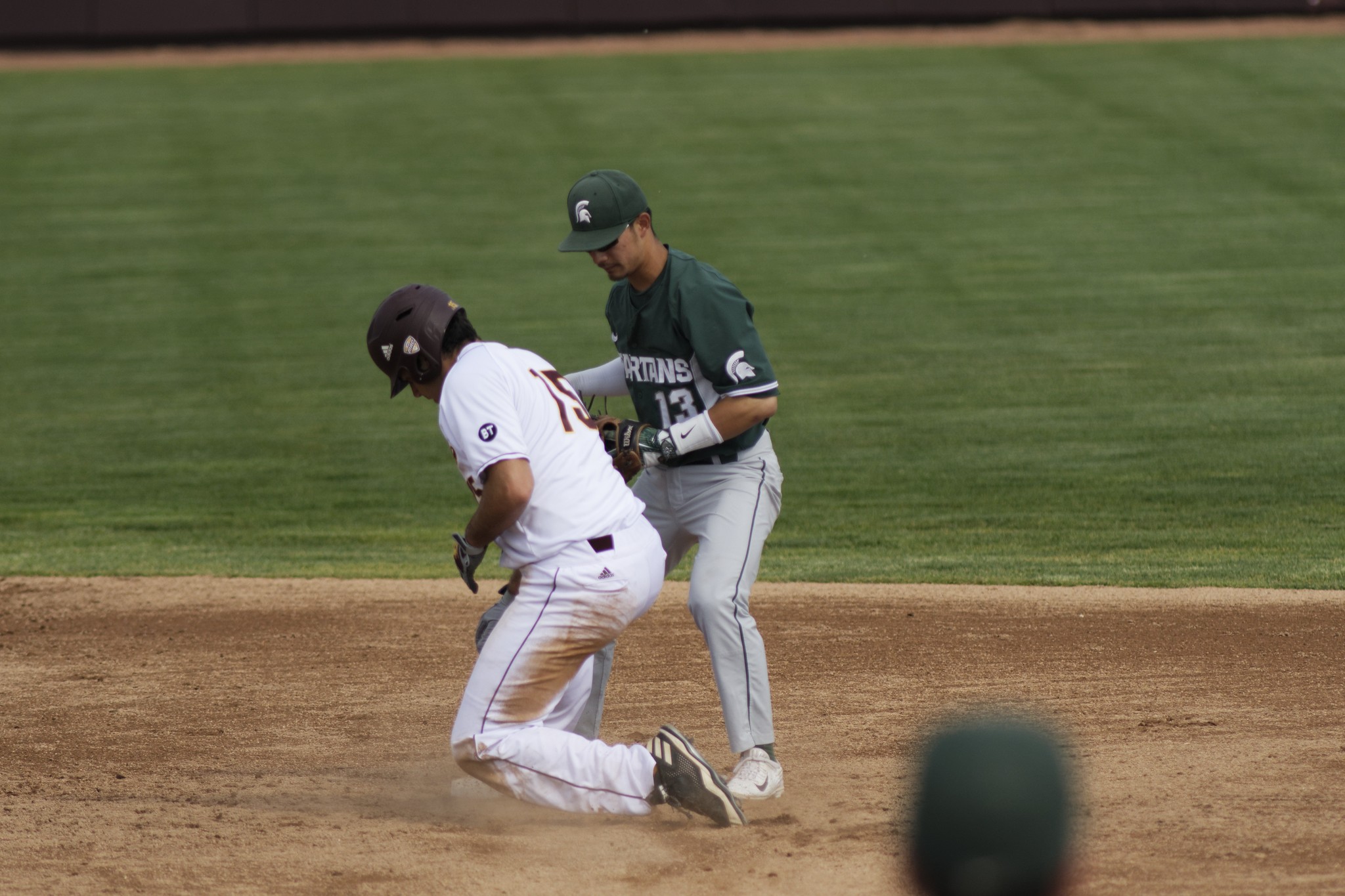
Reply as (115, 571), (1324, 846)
(592, 416), (652, 483)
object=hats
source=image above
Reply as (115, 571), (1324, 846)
(559, 169), (648, 252)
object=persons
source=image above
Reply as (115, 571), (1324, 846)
(907, 718), (1073, 896)
(365, 283), (751, 829)
(556, 168), (785, 802)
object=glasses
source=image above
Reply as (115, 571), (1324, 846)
(598, 222), (632, 253)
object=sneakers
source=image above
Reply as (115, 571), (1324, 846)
(643, 723), (748, 828)
(725, 746), (784, 801)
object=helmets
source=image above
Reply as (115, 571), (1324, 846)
(367, 283), (461, 399)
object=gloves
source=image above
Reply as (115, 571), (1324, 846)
(451, 533), (488, 595)
(474, 597), (508, 654)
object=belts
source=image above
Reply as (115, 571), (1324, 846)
(663, 454), (737, 468)
(588, 534), (614, 552)
